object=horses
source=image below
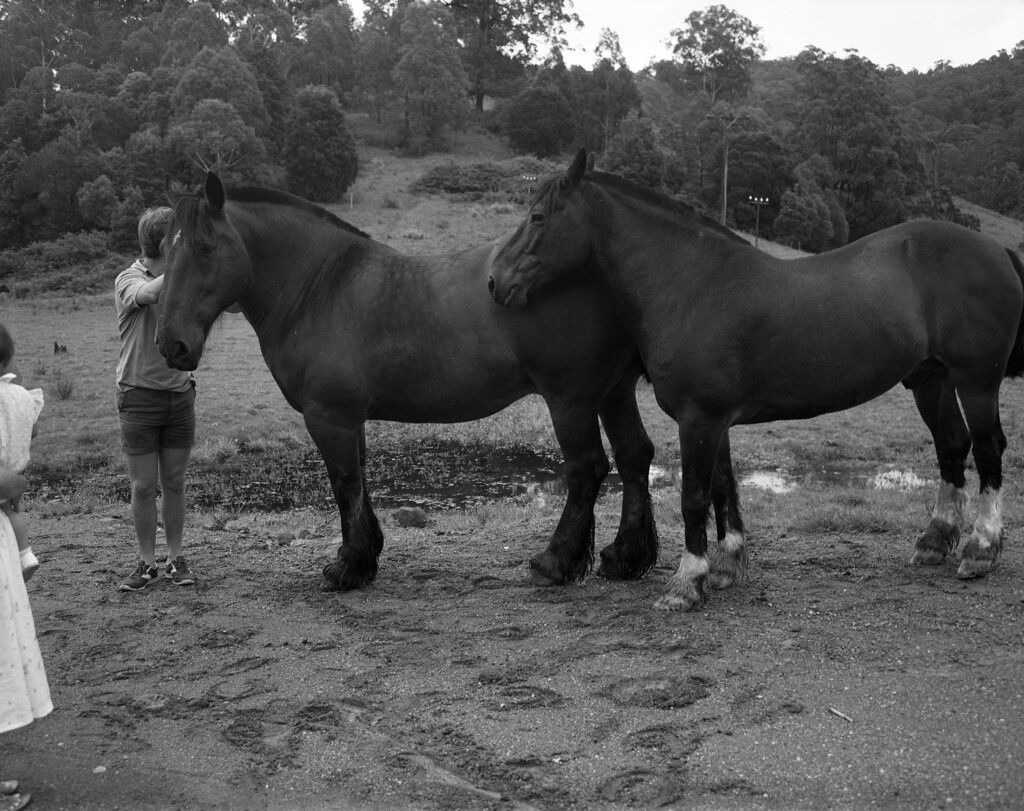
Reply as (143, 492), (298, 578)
(153, 169), (663, 593)
(486, 147), (1024, 613)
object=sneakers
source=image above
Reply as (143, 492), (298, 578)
(165, 556), (195, 585)
(120, 560), (159, 590)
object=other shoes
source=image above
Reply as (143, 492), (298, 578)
(0, 779), (32, 811)
(21, 552), (39, 583)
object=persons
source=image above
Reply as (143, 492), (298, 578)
(0, 324), (54, 809)
(114, 207), (199, 592)
